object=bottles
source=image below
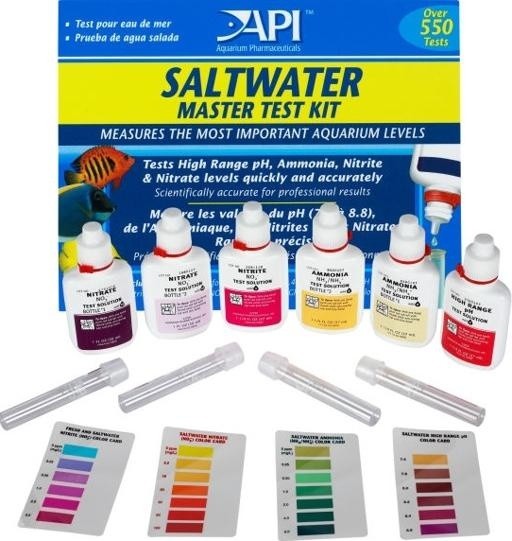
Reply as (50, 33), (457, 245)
(216, 201), (289, 334)
(296, 201), (366, 332)
(440, 235), (511, 372)
(410, 143), (461, 234)
(62, 220), (137, 356)
(141, 209), (215, 339)
(369, 214), (440, 349)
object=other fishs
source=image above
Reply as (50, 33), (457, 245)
(64, 145), (135, 190)
